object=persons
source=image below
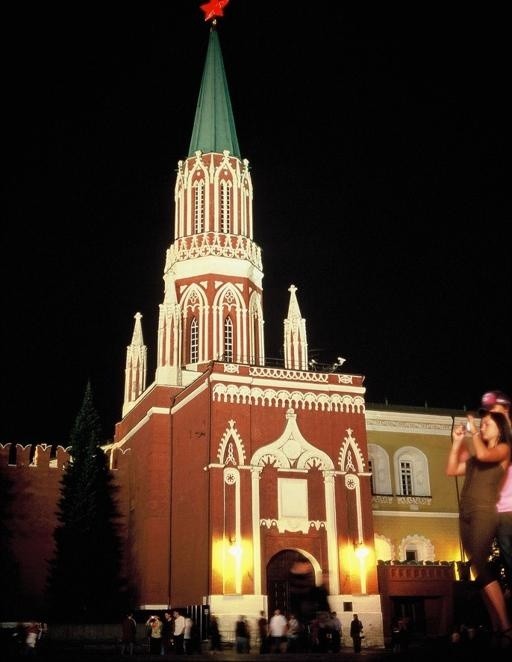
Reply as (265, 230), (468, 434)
(387, 612), (512, 662)
(118, 607), (365, 658)
(6, 617), (50, 662)
(487, 399), (511, 621)
(444, 409), (511, 650)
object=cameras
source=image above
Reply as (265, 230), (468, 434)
(462, 421), (470, 432)
